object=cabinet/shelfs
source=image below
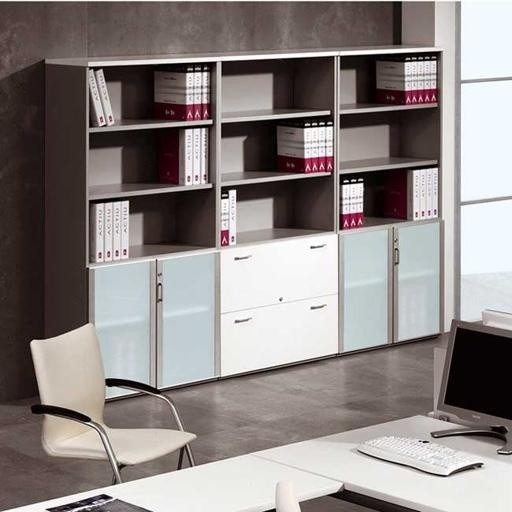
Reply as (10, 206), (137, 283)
(45, 53), (218, 401)
(335, 44), (444, 360)
(216, 49), (338, 381)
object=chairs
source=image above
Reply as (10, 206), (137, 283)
(29, 323), (196, 488)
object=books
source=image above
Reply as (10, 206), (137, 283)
(277, 119), (334, 173)
(383, 168), (438, 221)
(221, 189), (236, 246)
(89, 200), (129, 264)
(154, 64), (210, 121)
(157, 128), (208, 185)
(376, 55), (438, 105)
(88, 67), (114, 127)
(340, 176), (364, 229)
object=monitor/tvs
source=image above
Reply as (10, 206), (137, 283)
(481, 307), (511, 330)
(430, 319), (512, 455)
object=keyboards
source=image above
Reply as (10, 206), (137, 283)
(356, 435), (485, 476)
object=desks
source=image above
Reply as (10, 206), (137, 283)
(0, 453), (345, 512)
(252, 416), (510, 512)
